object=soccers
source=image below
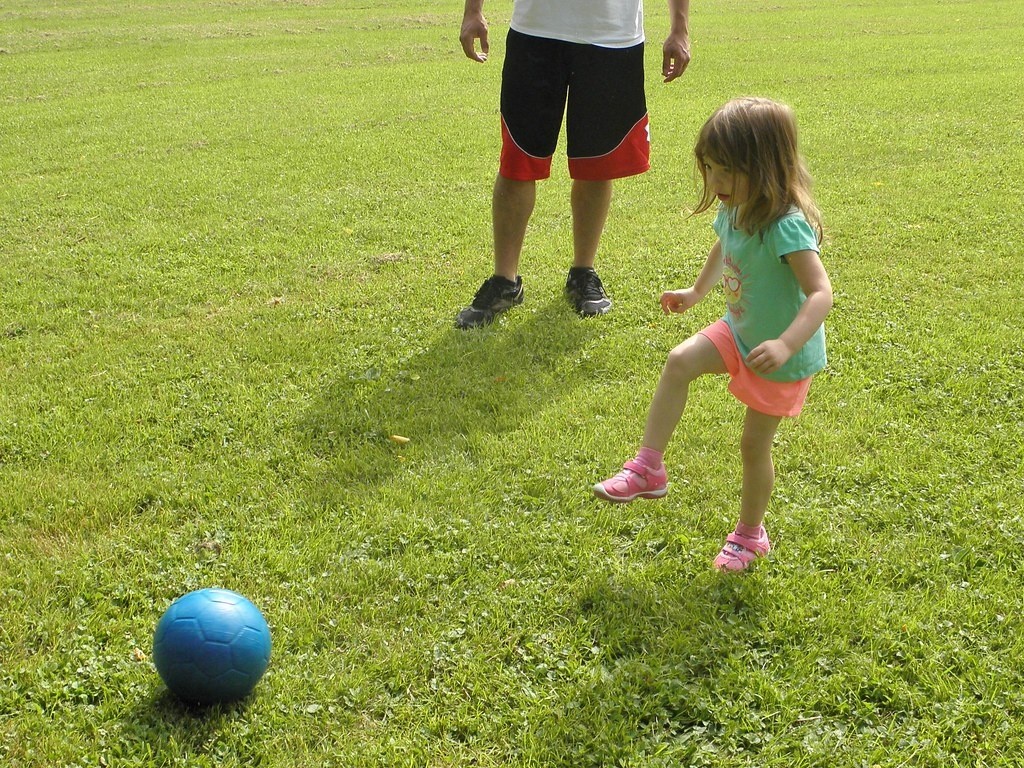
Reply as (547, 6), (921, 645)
(151, 588), (273, 708)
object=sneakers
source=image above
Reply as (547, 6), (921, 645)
(566, 268), (612, 315)
(455, 276), (525, 329)
(593, 458), (669, 503)
(712, 526), (770, 572)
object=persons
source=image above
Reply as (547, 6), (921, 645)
(453, 0), (690, 331)
(594, 98), (836, 575)
